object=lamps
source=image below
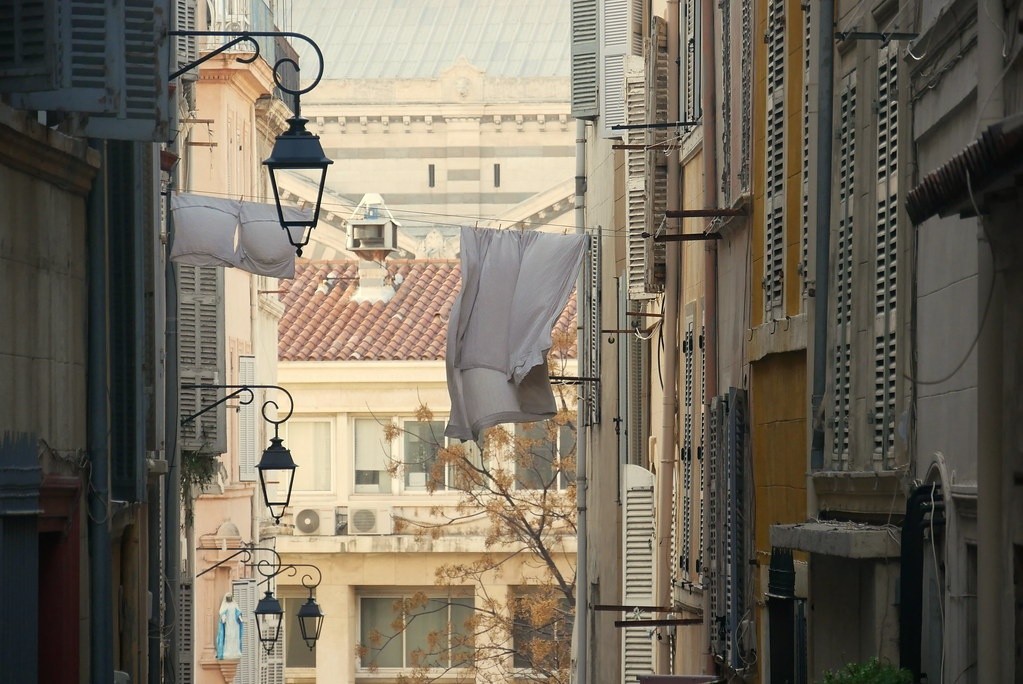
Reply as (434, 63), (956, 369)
(245, 563), (325, 653)
(180, 380), (299, 526)
(165, 29), (334, 258)
(197, 545), (285, 656)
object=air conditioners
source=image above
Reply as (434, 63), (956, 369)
(293, 490), (338, 535)
(348, 494), (390, 535)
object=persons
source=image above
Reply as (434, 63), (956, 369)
(216, 592), (243, 659)
